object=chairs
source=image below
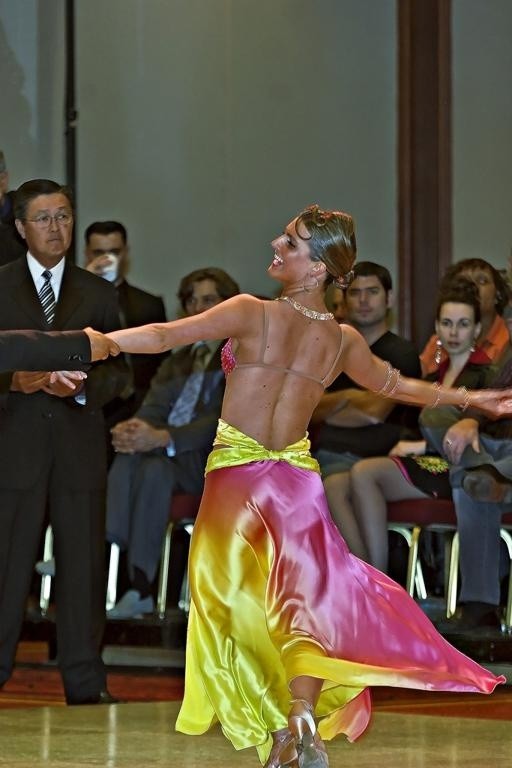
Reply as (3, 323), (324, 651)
(156, 492), (203, 619)
(385, 499), (454, 598)
(37, 496), (124, 614)
(445, 511), (512, 632)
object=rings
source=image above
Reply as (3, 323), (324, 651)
(446, 439), (453, 447)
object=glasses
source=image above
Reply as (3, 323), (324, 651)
(26, 212), (72, 227)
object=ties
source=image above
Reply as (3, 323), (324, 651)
(38, 271), (57, 323)
(167, 344), (210, 427)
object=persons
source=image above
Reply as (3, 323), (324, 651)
(417, 301), (512, 648)
(0, 154), (29, 264)
(0, 177), (122, 709)
(322, 272), (500, 575)
(50, 205), (511, 768)
(85, 222), (172, 608)
(34, 267), (238, 618)
(417, 255), (512, 379)
(315, 259), (423, 485)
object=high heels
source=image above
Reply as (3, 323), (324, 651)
(264, 729), (299, 768)
(287, 700), (328, 768)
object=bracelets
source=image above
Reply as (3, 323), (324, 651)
(457, 384), (471, 413)
(386, 368), (402, 398)
(431, 379), (444, 410)
(375, 358), (393, 395)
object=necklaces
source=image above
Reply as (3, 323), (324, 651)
(276, 292), (336, 322)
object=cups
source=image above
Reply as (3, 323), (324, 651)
(101, 253), (120, 282)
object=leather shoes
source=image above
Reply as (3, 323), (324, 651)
(87, 690), (126, 704)
(433, 603), (495, 643)
(105, 589), (157, 618)
